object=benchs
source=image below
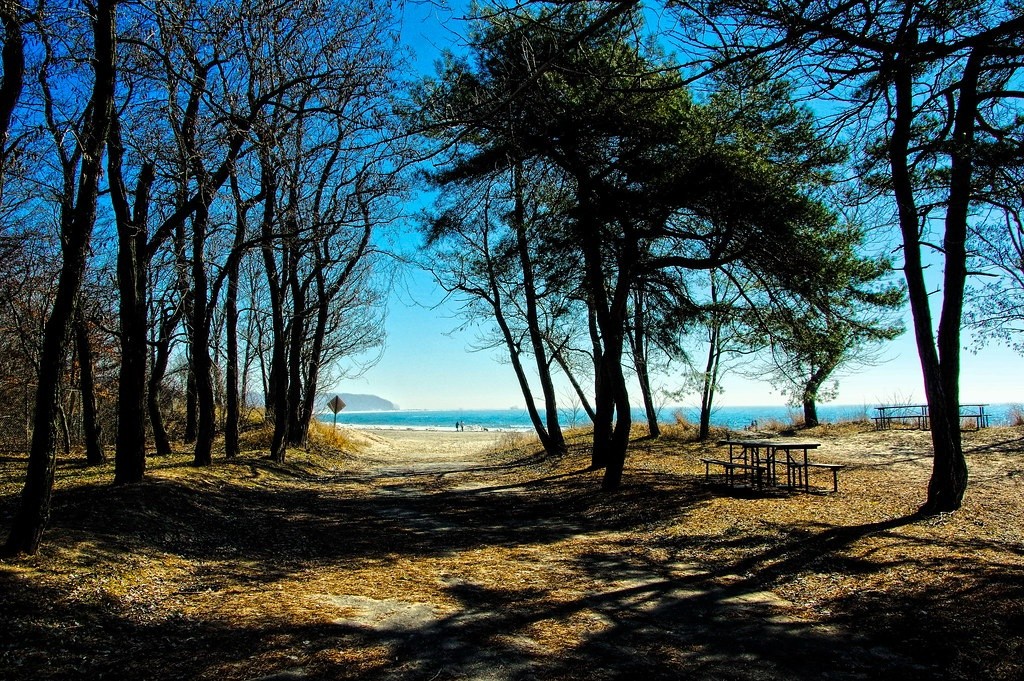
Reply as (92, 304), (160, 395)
(871, 414), (991, 431)
(700, 457), (766, 491)
(762, 458), (848, 492)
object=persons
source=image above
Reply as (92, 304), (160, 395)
(456, 422), (459, 431)
(744, 420), (758, 433)
(484, 428), (488, 432)
(460, 421), (464, 432)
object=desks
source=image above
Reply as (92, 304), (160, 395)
(717, 440), (821, 493)
(874, 404), (927, 430)
(927, 403), (989, 432)
